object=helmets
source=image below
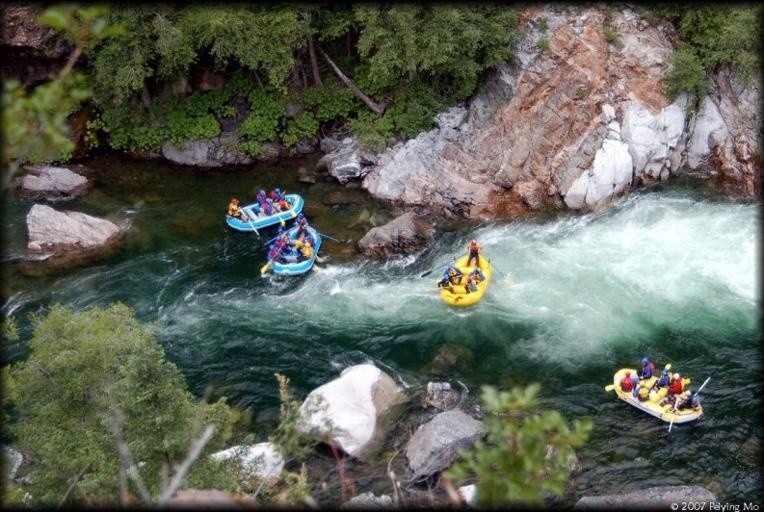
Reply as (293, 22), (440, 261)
(266, 198), (272, 204)
(260, 190), (266, 196)
(278, 228), (284, 234)
(281, 195), (286, 200)
(275, 188), (280, 194)
(231, 198), (237, 204)
(299, 212), (305, 219)
(472, 240), (476, 245)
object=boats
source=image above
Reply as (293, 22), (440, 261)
(227, 192), (304, 232)
(267, 224), (322, 277)
(611, 367), (706, 424)
(438, 254), (493, 306)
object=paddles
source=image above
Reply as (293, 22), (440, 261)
(605, 384), (620, 391)
(289, 205), (297, 218)
(272, 206), (286, 226)
(421, 252), (468, 277)
(693, 364), (717, 398)
(239, 208), (264, 249)
(260, 243), (285, 273)
(662, 408), (677, 440)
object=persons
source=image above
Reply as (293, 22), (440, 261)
(228, 188), (314, 264)
(438, 240), (485, 294)
(620, 357), (699, 414)
